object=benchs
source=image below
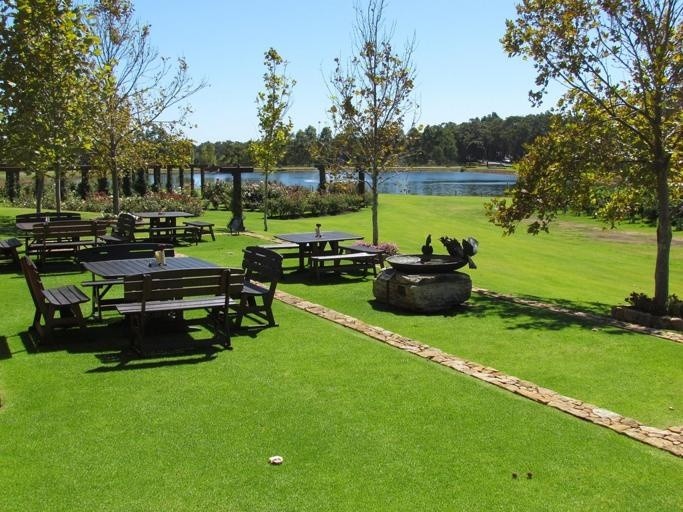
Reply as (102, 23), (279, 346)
(260, 244), (340, 270)
(205, 246), (284, 334)
(1, 212), (136, 275)
(114, 268), (245, 366)
(21, 256), (91, 347)
(310, 253), (384, 281)
(98, 212), (216, 246)
(81, 245), (183, 322)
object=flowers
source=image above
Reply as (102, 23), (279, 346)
(355, 242), (399, 257)
